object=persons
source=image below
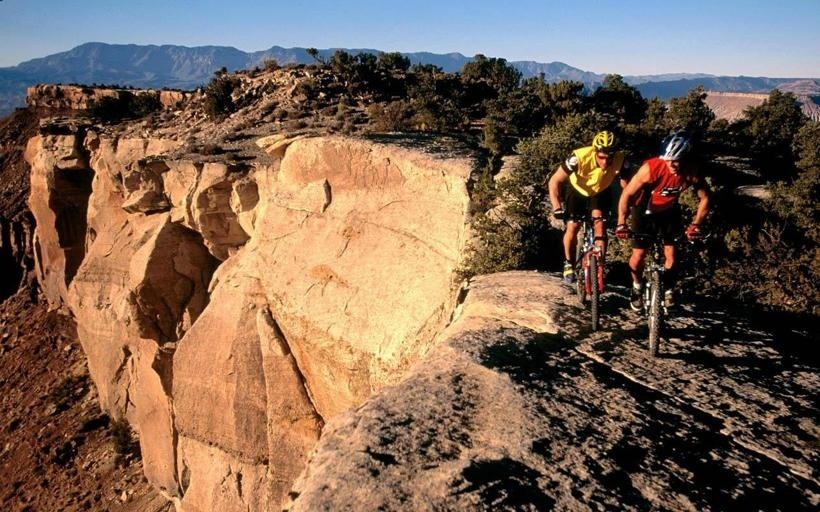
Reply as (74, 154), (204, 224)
(615, 129), (713, 313)
(546, 129), (634, 285)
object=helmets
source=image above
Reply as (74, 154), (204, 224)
(659, 131), (691, 162)
(592, 131), (620, 153)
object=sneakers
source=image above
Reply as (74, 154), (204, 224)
(629, 287), (644, 315)
(564, 259), (575, 285)
(664, 289), (675, 308)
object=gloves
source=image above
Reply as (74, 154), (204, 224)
(615, 224), (633, 240)
(550, 207), (567, 232)
(685, 224), (706, 241)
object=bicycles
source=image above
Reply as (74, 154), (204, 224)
(615, 227), (706, 357)
(554, 209), (619, 330)
